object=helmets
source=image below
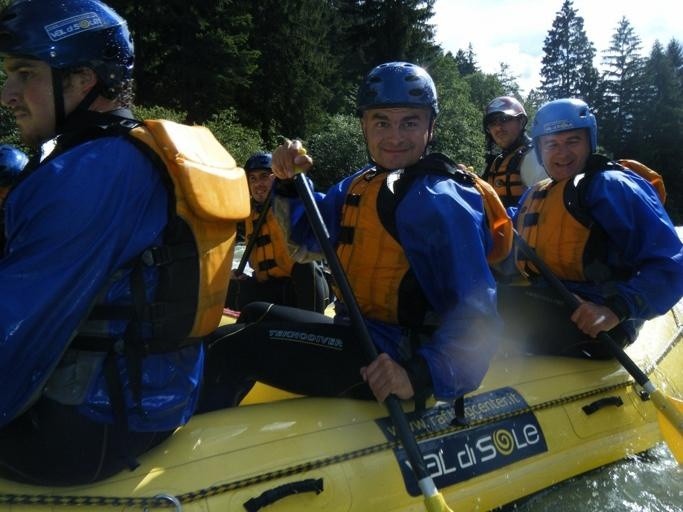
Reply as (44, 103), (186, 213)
(1, 1), (135, 88)
(1, 143), (32, 179)
(357, 61), (439, 120)
(244, 153), (272, 172)
(483, 96), (528, 131)
(532, 98), (597, 166)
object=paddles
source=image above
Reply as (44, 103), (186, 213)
(513, 229), (683, 466)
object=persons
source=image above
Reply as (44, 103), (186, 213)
(477, 97), (547, 213)
(223, 151), (331, 313)
(200, 62), (504, 414)
(0, 0), (251, 486)
(512, 99), (683, 367)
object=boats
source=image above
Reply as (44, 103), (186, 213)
(1, 297), (683, 512)
